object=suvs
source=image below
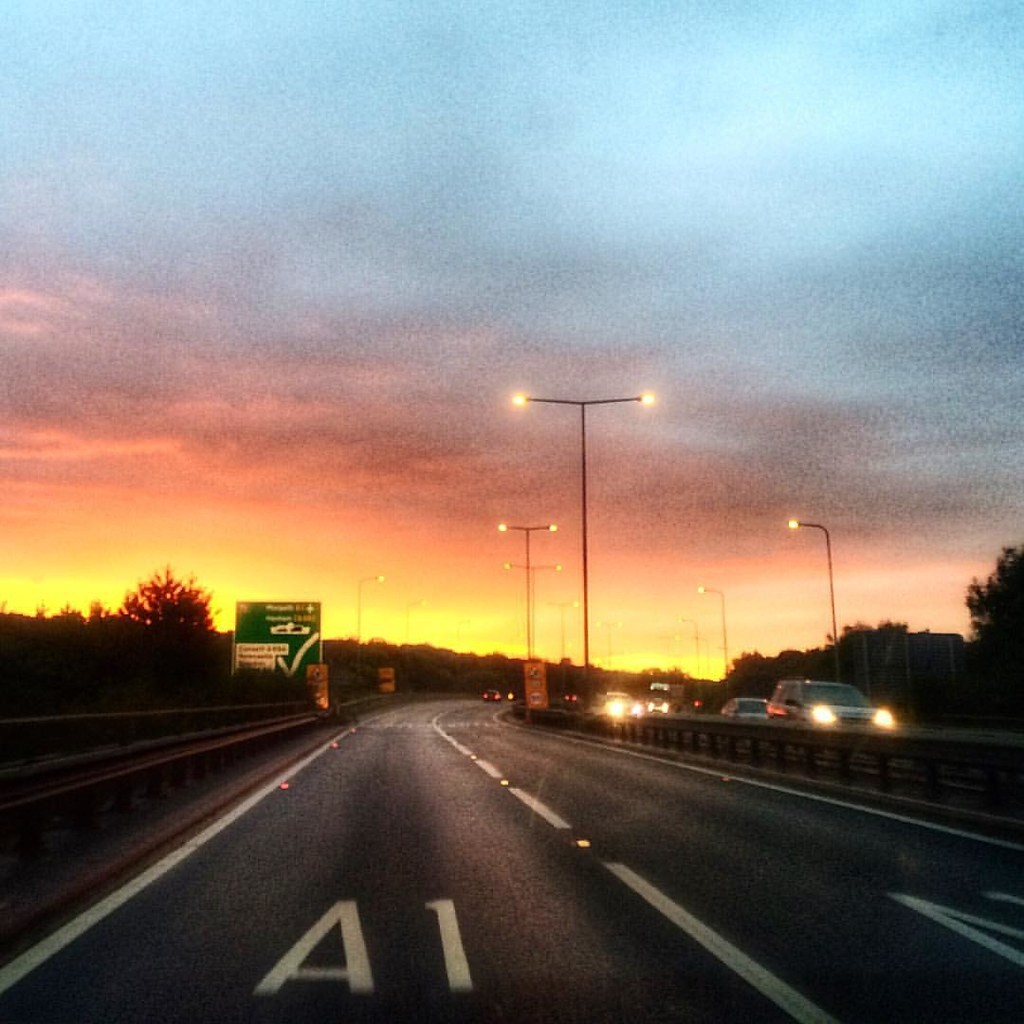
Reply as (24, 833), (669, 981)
(767, 678), (885, 728)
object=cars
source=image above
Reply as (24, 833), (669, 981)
(719, 696), (768, 724)
(592, 682), (707, 715)
(482, 687), (586, 706)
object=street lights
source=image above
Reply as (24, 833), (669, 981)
(500, 518), (557, 661)
(513, 390), (656, 691)
(352, 571), (386, 693)
(697, 585), (731, 693)
(505, 557), (565, 662)
(789, 519), (843, 683)
